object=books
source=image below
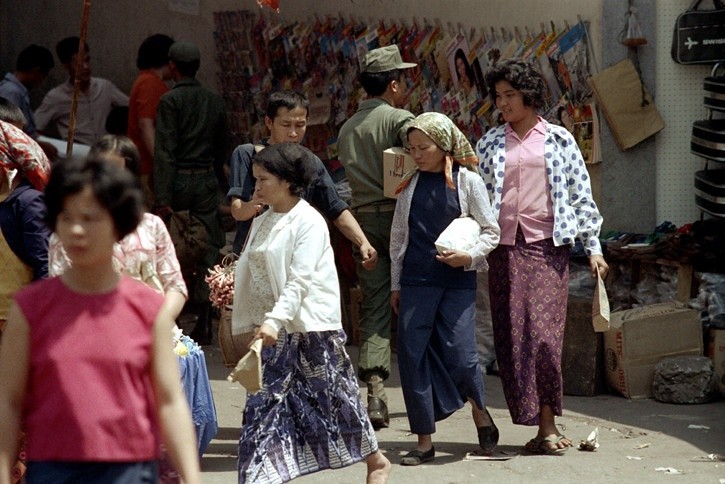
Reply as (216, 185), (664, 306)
(211, 8), (607, 169)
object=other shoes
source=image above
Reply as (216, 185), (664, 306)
(197, 311), (212, 345)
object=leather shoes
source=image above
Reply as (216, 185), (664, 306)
(367, 397), (390, 428)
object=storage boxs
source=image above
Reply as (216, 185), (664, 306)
(561, 299), (725, 401)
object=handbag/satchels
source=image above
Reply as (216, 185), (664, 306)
(219, 311), (260, 368)
(670, 0), (725, 65)
(167, 209), (210, 264)
(591, 262), (610, 333)
(434, 215), (482, 258)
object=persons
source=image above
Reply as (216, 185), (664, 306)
(1, 43), (58, 162)
(469, 59), (609, 457)
(33, 36), (133, 147)
(151, 40), (239, 346)
(336, 44), (420, 428)
(228, 88), (379, 299)
(0, 121), (53, 484)
(390, 109), (503, 464)
(0, 96), (27, 132)
(232, 142), (393, 484)
(125, 32), (177, 225)
(48, 134), (187, 337)
(0, 156), (203, 484)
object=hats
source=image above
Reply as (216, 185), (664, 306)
(363, 44), (418, 73)
(167, 41), (200, 63)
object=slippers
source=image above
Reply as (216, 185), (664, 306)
(537, 434), (570, 455)
(473, 408), (500, 453)
(524, 437), (542, 452)
(399, 446), (436, 466)
